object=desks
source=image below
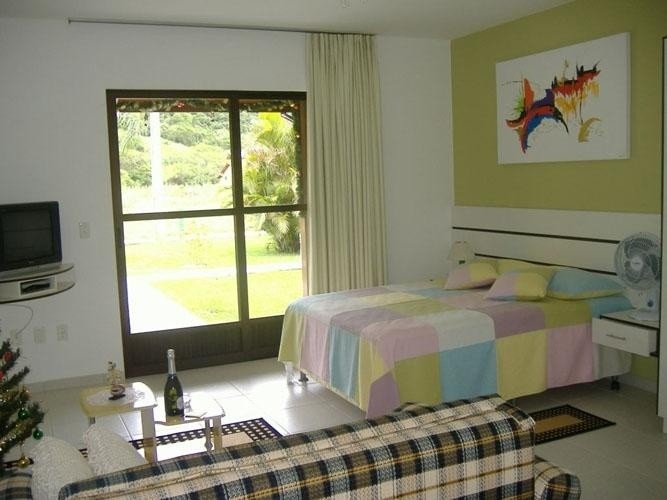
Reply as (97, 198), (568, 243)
(589, 308), (658, 392)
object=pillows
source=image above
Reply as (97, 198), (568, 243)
(28, 435), (93, 499)
(482, 265), (553, 303)
(81, 420), (150, 476)
(440, 253), (499, 292)
(546, 269), (626, 303)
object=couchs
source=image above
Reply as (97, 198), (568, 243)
(0, 392), (581, 498)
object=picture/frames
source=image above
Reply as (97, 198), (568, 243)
(494, 31), (631, 167)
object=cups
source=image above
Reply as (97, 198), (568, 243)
(182, 392), (192, 415)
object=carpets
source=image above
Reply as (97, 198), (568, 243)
(78, 417), (284, 465)
(525, 402), (616, 446)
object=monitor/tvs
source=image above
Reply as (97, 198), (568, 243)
(0, 201), (63, 275)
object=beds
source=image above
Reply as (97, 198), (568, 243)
(276, 202), (661, 421)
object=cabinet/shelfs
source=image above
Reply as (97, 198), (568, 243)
(0, 259), (78, 305)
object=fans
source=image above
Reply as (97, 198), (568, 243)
(611, 229), (662, 323)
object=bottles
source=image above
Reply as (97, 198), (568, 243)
(163, 348), (183, 416)
